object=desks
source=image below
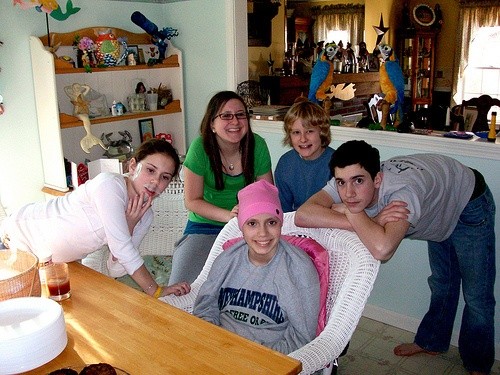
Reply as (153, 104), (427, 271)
(20, 260), (303, 375)
(260, 73), (384, 112)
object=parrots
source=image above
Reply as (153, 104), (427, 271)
(377, 43), (405, 125)
(308, 44), (342, 105)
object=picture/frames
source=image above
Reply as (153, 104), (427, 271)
(138, 118), (155, 144)
(125, 45), (139, 64)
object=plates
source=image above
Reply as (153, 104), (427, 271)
(0, 297), (67, 375)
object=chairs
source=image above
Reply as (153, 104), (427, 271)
(82, 183), (199, 295)
(460, 95), (500, 134)
(154, 209), (381, 375)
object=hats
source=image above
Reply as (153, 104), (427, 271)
(238, 179), (283, 230)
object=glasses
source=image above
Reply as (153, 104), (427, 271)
(212, 113), (247, 120)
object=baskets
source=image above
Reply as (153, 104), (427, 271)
(0, 249), (41, 302)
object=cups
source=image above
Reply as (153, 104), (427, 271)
(334, 61), (342, 73)
(38, 255), (53, 285)
(352, 63), (359, 73)
(44, 262), (71, 302)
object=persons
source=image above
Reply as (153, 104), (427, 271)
(193, 180), (319, 355)
(167, 92), (274, 294)
(1, 138), (191, 299)
(294, 139), (495, 374)
(275, 100), (338, 213)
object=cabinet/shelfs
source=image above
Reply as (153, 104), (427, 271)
(396, 0), (435, 119)
(30, 26), (188, 195)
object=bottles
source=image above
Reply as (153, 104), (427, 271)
(112, 99), (124, 117)
(487, 112), (497, 142)
(146, 90), (158, 111)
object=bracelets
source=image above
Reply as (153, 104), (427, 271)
(143, 281), (156, 293)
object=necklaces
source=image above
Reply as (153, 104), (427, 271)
(223, 149), (240, 171)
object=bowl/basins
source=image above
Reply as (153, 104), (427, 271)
(474, 131), (489, 139)
